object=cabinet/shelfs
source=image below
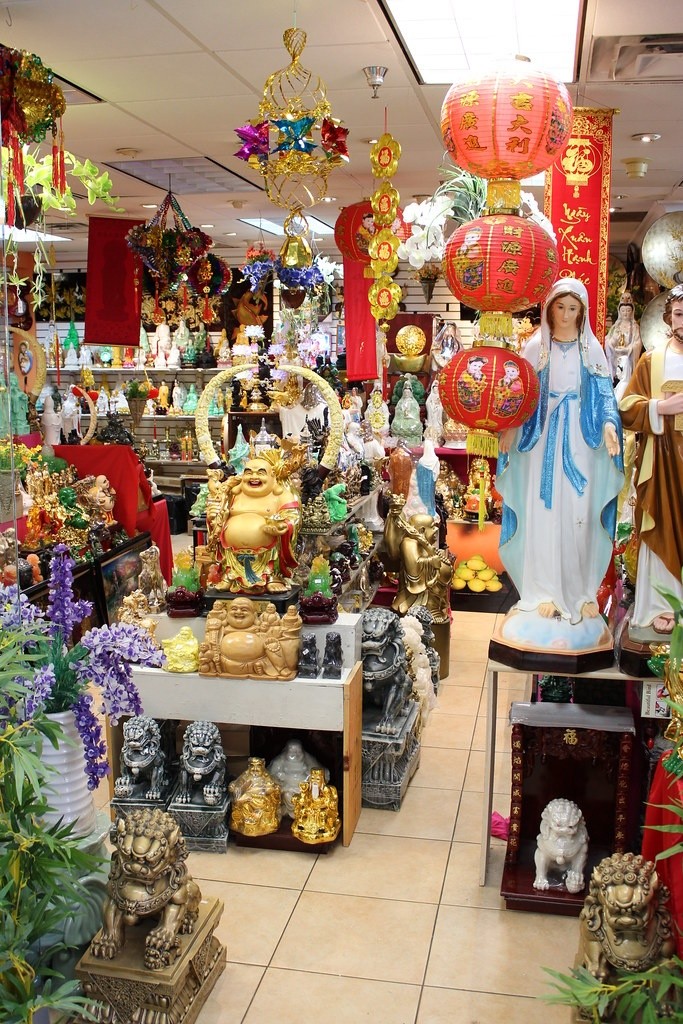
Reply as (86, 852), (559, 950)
(108, 613), (363, 848)
(47, 365), (230, 470)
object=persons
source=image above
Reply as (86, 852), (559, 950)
(161, 625), (198, 673)
(229, 741), (341, 844)
(340, 317), (491, 510)
(199, 598), (301, 679)
(207, 458), (328, 592)
(499, 275), (682, 618)
(117, 589), (168, 650)
(16, 460), (170, 588)
(390, 514), (458, 625)
(39, 315), (261, 447)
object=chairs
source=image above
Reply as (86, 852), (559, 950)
(15, 433), (174, 584)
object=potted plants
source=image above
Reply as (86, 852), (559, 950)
(126, 379), (148, 427)
(0, 142), (126, 230)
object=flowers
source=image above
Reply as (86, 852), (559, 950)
(396, 147), (557, 271)
(235, 246), (344, 294)
(0, 544), (165, 790)
(0, 434), (44, 471)
(408, 264), (444, 282)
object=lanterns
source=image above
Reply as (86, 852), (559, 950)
(442, 209), (561, 335)
(437, 52), (575, 208)
(437, 338), (542, 530)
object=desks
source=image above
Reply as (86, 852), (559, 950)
(480, 656), (662, 887)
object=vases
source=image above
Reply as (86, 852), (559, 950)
(0, 470), (20, 515)
(30, 707), (102, 838)
(420, 279), (437, 305)
(278, 283), (309, 309)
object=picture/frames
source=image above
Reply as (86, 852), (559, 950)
(19, 558), (102, 650)
(96, 531), (152, 622)
